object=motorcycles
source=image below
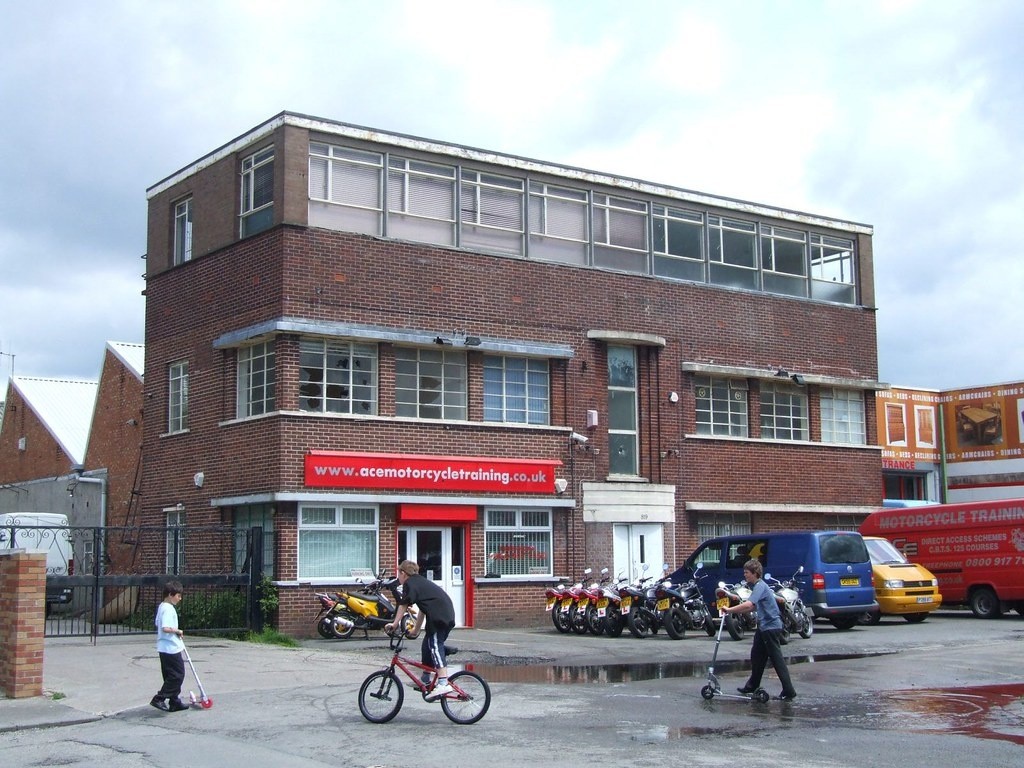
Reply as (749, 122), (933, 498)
(763, 566), (813, 644)
(543, 567), (716, 641)
(715, 579), (758, 640)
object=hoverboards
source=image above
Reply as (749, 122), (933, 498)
(176, 634), (213, 708)
(700, 610), (769, 703)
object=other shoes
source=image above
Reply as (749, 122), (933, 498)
(150, 695), (169, 711)
(424, 684), (454, 700)
(168, 701), (189, 711)
(773, 691), (798, 701)
(736, 685), (755, 693)
(406, 678), (432, 688)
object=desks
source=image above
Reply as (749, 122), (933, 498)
(960, 408), (997, 442)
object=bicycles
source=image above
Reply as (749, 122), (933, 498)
(358, 627), (491, 725)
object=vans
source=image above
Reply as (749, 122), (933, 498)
(858, 498), (1024, 618)
(657, 531), (879, 629)
(749, 537), (943, 625)
(0, 511), (76, 617)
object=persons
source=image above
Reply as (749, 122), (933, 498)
(722, 557), (796, 701)
(385, 561), (455, 701)
(149, 580), (189, 713)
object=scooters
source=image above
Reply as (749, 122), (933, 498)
(312, 568), (420, 640)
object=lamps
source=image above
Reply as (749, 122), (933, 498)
(434, 335), (453, 347)
(791, 374), (805, 387)
(773, 366), (789, 379)
(464, 337), (481, 346)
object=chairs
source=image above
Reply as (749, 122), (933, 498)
(732, 547), (753, 570)
(961, 405), (998, 444)
(759, 546), (766, 567)
